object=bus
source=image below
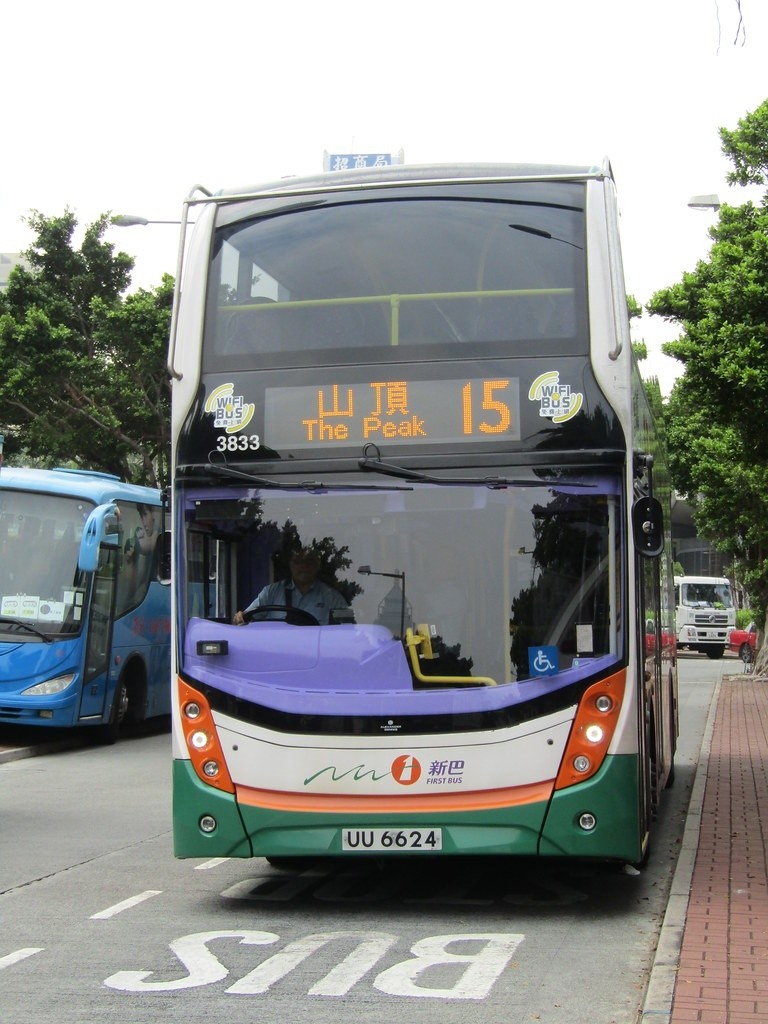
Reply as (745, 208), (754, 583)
(0, 465), (215, 745)
(149, 157), (677, 873)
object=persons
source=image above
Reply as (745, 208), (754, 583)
(528, 528), (595, 650)
(232, 535), (347, 626)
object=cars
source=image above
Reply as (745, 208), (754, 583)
(729, 622), (758, 664)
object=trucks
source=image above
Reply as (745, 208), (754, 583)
(673, 573), (743, 659)
